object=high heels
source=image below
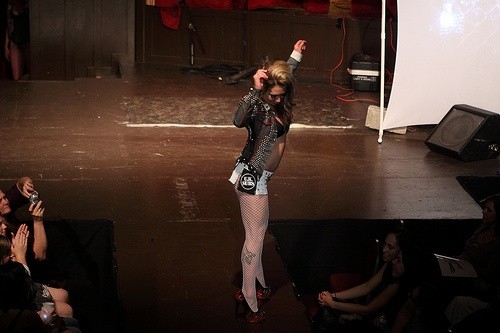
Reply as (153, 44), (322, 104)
(235, 290), (267, 324)
(255, 277), (272, 300)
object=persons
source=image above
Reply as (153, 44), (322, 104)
(317, 226), (407, 333)
(0, 176), (81, 333)
(228, 40), (306, 325)
(390, 194), (500, 333)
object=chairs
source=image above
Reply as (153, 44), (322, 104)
(328, 237), (382, 288)
(387, 287), (423, 333)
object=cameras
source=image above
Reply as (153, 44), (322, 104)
(29, 191), (40, 208)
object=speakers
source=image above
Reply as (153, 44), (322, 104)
(424, 103), (500, 162)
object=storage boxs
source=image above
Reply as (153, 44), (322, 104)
(347, 61), (379, 93)
